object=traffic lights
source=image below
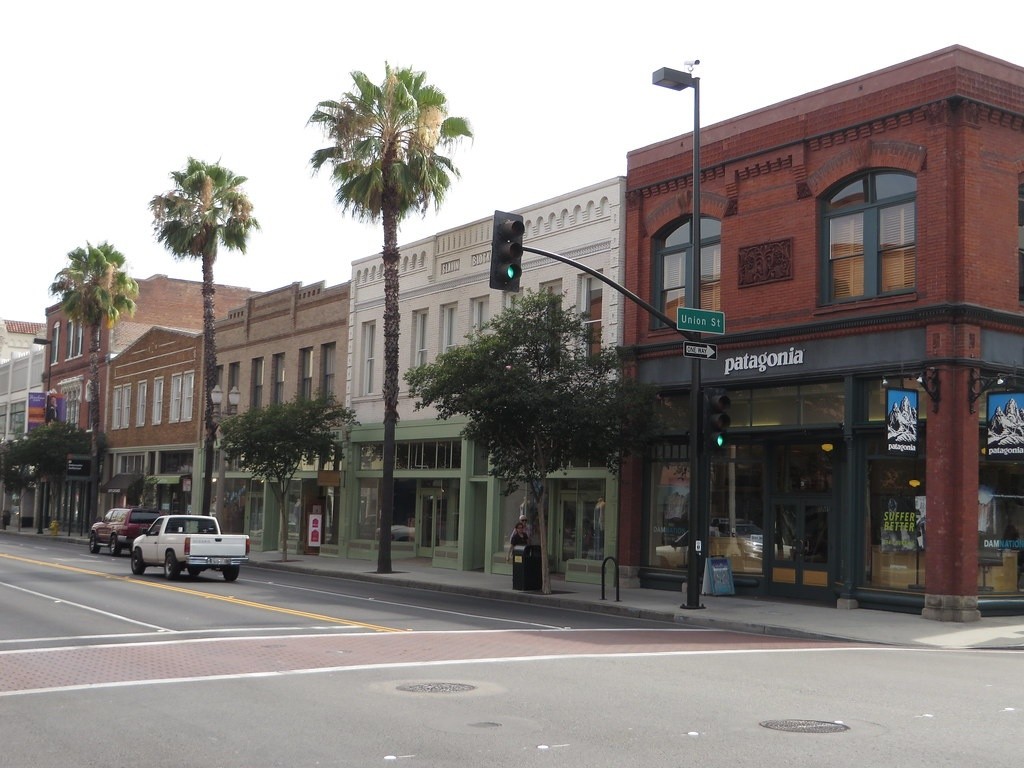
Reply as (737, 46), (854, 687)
(701, 387), (730, 453)
(489, 210), (524, 293)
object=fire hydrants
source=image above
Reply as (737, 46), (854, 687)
(50, 519), (60, 536)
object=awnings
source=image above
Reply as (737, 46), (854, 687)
(100, 474), (191, 494)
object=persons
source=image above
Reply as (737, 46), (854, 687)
(506, 523), (528, 561)
(46, 396), (58, 422)
(510, 515), (533, 557)
(593, 498), (605, 560)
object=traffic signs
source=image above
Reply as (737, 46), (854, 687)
(682, 341), (718, 360)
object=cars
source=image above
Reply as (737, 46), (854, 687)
(656, 517), (797, 567)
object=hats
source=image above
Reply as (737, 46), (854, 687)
(519, 515), (527, 520)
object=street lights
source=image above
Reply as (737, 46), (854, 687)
(650, 67), (709, 610)
(33, 337), (53, 534)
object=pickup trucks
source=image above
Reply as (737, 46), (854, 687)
(129, 513), (252, 581)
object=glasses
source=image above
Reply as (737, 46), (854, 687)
(521, 519), (527, 522)
(517, 527), (524, 529)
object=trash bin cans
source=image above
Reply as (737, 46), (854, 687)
(2, 510), (11, 526)
(512, 543), (543, 591)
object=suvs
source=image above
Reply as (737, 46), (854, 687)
(87, 507), (168, 557)
